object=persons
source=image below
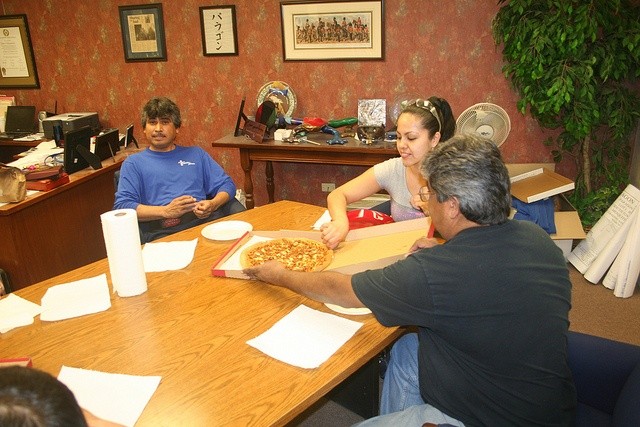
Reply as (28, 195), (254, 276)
(321, 94), (457, 251)
(295, 17), (369, 42)
(239, 132), (578, 426)
(1, 363), (128, 427)
(113, 97), (248, 241)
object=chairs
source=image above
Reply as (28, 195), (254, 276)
(113, 170), (246, 231)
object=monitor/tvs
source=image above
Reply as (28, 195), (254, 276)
(4, 104), (36, 135)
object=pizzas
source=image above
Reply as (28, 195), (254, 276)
(240, 238), (333, 272)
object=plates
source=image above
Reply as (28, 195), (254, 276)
(200, 220), (253, 242)
(256, 81), (297, 119)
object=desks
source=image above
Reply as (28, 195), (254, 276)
(0, 129), (149, 290)
(212, 130), (400, 210)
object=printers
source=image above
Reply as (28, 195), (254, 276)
(41, 111), (100, 141)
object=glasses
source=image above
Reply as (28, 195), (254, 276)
(401, 98), (442, 132)
(419, 186), (438, 202)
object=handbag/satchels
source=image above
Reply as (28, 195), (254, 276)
(0, 163), (27, 204)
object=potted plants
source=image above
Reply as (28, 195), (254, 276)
(490, 0), (640, 244)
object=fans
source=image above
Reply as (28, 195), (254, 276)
(454, 104), (512, 148)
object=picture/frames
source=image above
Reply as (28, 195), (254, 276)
(119, 3), (167, 63)
(199, 4), (239, 56)
(0, 14), (40, 88)
(279, 1), (385, 63)
